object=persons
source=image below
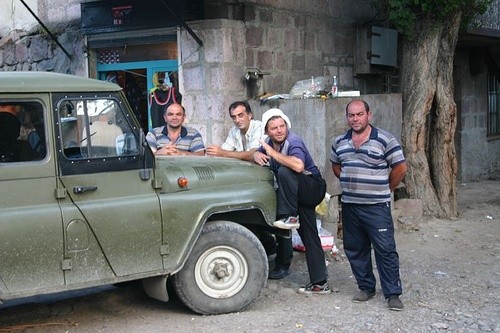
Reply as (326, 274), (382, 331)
(329, 99), (408, 311)
(206, 101), (293, 280)
(145, 102), (206, 156)
(253, 107), (332, 295)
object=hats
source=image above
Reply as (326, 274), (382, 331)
(261, 108), (291, 135)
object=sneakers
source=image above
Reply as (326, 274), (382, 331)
(274, 215), (300, 230)
(386, 296), (403, 311)
(266, 244), (278, 256)
(352, 290), (375, 303)
(268, 265), (290, 280)
(297, 282), (332, 295)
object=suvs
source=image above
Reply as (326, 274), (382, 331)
(0, 71), (278, 316)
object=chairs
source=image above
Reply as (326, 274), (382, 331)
(0, 111), (36, 161)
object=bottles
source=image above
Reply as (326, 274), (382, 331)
(310, 76), (316, 98)
(332, 76), (338, 98)
